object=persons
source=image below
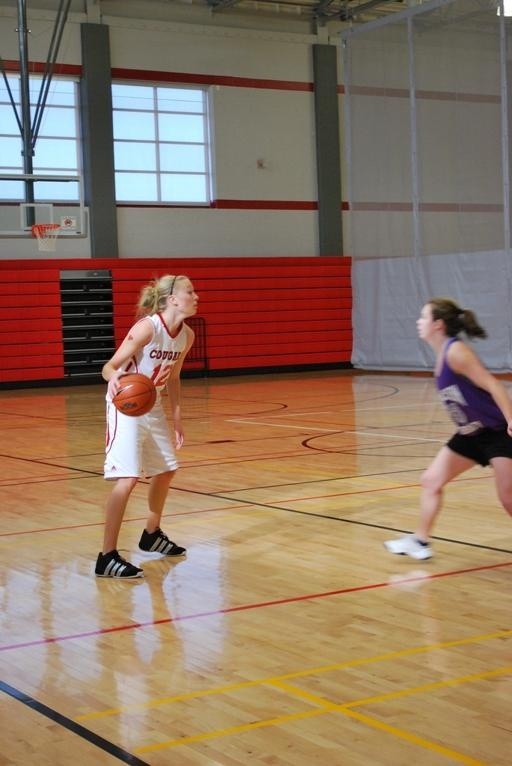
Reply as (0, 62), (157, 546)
(94, 275), (200, 579)
(382, 298), (511, 560)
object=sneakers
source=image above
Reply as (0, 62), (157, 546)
(383, 535), (432, 560)
(139, 529), (186, 556)
(95, 550), (144, 579)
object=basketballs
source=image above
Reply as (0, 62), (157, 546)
(112, 374), (156, 416)
